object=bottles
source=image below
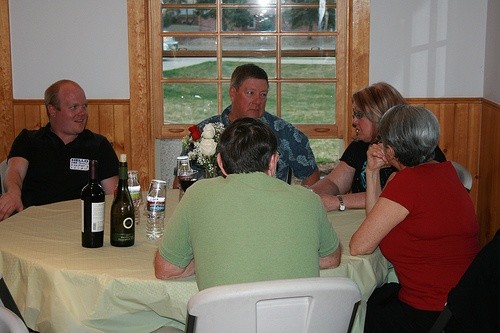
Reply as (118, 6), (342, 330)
(127, 172), (142, 224)
(146, 180), (168, 239)
(178, 161), (191, 202)
(81, 161), (105, 248)
(110, 154), (135, 247)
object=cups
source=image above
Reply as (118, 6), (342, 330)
(178, 170), (198, 191)
(147, 181), (166, 202)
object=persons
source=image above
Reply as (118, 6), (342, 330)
(154, 117), (341, 291)
(173, 64), (320, 187)
(0, 79), (120, 220)
(349, 105), (480, 333)
(307, 83), (446, 213)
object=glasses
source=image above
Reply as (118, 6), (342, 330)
(352, 111), (369, 120)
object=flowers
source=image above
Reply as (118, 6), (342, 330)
(181, 122), (225, 179)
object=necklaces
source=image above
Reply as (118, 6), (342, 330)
(228, 111), (231, 122)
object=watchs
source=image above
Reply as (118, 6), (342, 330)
(336, 194), (347, 211)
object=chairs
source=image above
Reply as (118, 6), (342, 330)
(155, 138), (184, 189)
(431, 230), (500, 333)
(186, 277), (362, 333)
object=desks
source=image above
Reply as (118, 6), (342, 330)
(0, 189), (399, 333)
